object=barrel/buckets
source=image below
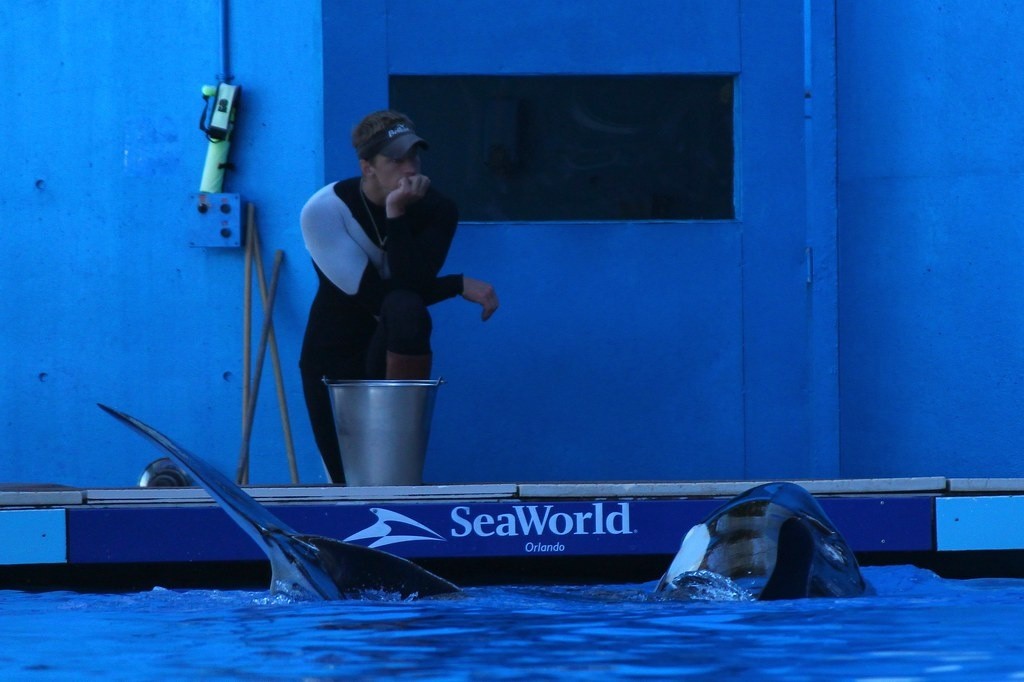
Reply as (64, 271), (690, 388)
(323, 376), (445, 485)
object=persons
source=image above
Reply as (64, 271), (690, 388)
(298, 109), (500, 483)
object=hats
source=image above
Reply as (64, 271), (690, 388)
(357, 124), (429, 165)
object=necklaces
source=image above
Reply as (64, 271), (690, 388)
(358, 179), (390, 271)
